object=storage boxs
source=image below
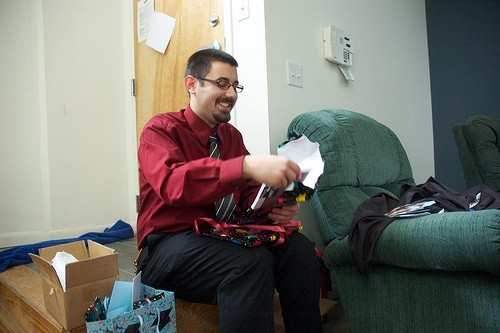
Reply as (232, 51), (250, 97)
(28, 239), (120, 330)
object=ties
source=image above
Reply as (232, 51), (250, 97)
(209, 132), (237, 224)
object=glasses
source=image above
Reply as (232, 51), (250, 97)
(184, 74), (244, 93)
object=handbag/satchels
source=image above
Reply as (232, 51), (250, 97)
(85, 282), (177, 333)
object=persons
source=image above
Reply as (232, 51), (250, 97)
(136, 49), (322, 333)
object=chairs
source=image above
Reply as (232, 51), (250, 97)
(455, 116), (500, 195)
(279, 111), (500, 333)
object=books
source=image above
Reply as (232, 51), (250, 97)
(247, 170), (309, 220)
